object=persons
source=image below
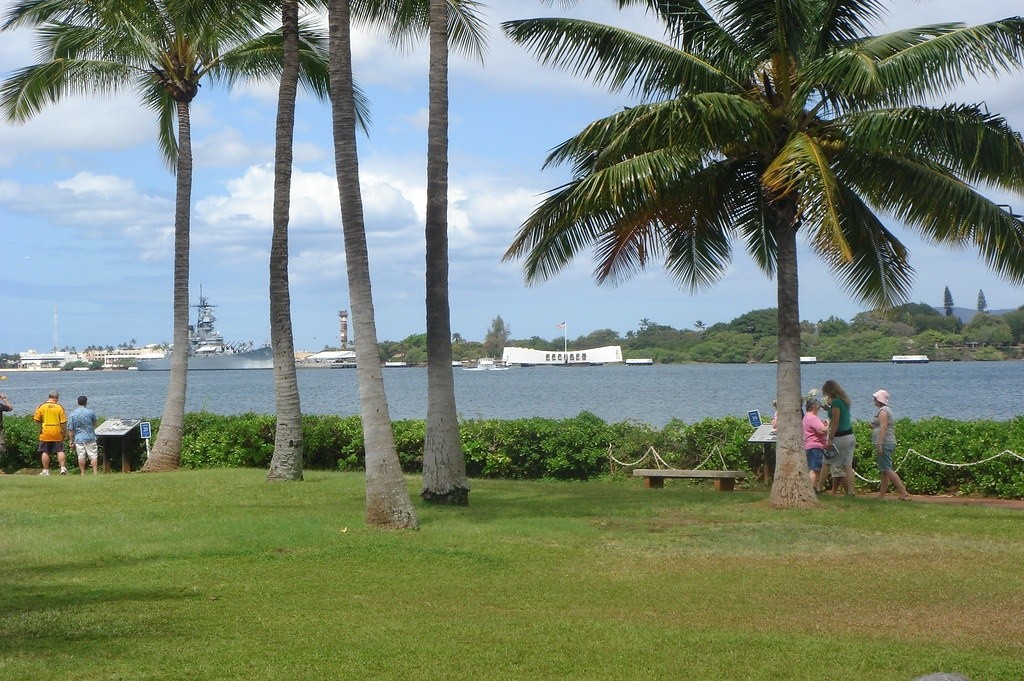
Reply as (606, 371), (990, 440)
(772, 400), (778, 429)
(0, 393), (13, 474)
(33, 389), (69, 477)
(872, 390), (908, 501)
(803, 380), (856, 497)
(69, 395), (98, 479)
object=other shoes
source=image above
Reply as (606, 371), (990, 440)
(40, 470), (49, 476)
(899, 494), (911, 501)
(60, 467), (67, 474)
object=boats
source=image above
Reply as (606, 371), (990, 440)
(135, 282), (275, 372)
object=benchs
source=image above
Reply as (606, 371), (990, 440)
(633, 467), (746, 492)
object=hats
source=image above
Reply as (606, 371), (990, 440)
(873, 389), (888, 405)
